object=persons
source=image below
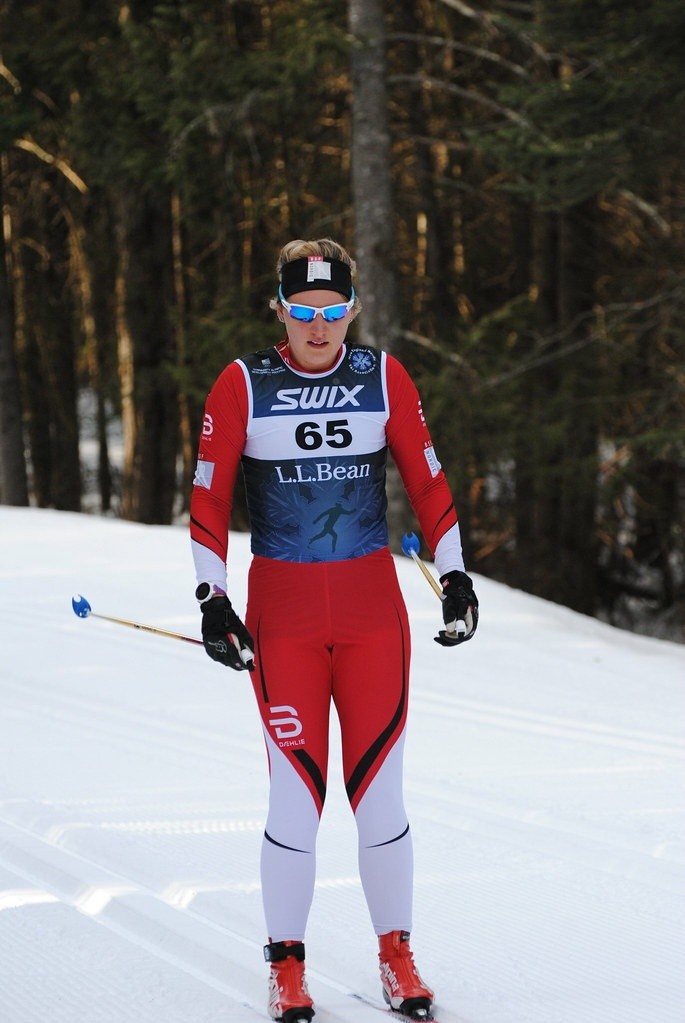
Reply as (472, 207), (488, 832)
(190, 240), (480, 1023)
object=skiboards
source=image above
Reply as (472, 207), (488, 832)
(347, 989), (442, 1023)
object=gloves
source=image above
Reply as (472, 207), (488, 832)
(200, 595), (256, 671)
(433, 570), (479, 647)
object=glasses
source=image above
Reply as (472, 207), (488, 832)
(279, 283), (355, 322)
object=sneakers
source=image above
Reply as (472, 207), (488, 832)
(263, 940), (315, 1020)
(377, 930), (434, 1013)
